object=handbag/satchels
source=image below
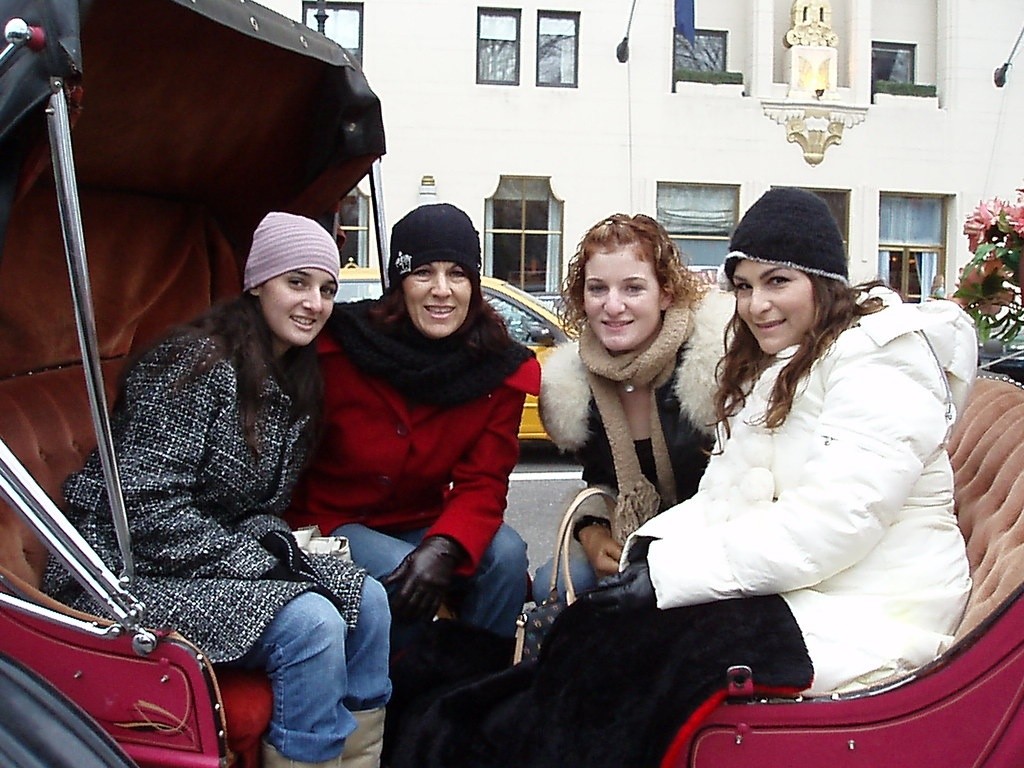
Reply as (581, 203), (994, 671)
(512, 484), (617, 674)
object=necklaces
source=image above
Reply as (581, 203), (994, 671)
(624, 384), (634, 393)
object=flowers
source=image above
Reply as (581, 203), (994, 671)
(954, 184), (1024, 356)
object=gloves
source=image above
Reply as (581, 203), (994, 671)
(583, 559), (657, 619)
(382, 537), (466, 623)
(259, 562), (342, 610)
(259, 531), (322, 580)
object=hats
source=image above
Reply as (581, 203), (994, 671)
(724, 186), (849, 285)
(388, 203), (482, 281)
(243, 211), (341, 298)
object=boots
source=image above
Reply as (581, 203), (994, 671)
(341, 705), (386, 768)
(262, 739), (341, 768)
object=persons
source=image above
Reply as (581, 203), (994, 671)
(44, 188), (978, 768)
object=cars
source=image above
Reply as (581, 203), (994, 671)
(332, 268), (588, 467)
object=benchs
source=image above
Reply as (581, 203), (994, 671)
(659, 366), (1024, 768)
(0, 189), (276, 768)
